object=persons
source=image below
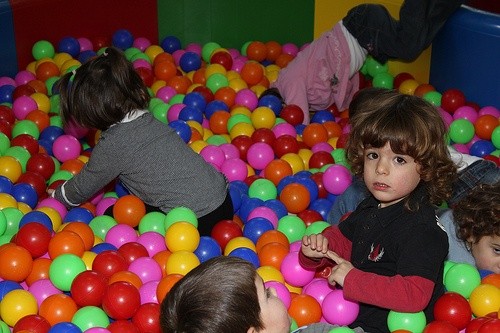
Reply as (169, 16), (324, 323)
(158, 256), (369, 333)
(327, 177), (500, 273)
(257, 0), (466, 126)
(298, 93), (460, 333)
(45, 46), (234, 238)
(326, 87), (499, 226)
